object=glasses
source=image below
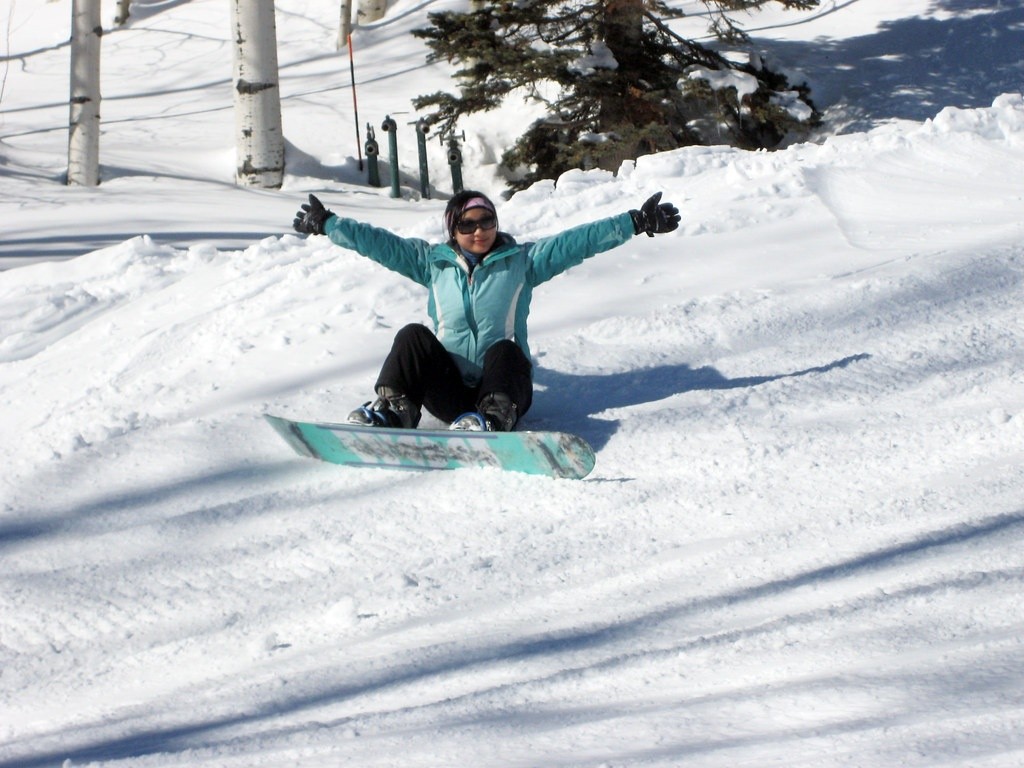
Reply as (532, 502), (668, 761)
(455, 216), (496, 235)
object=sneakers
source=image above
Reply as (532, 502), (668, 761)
(448, 392), (517, 432)
(348, 385), (422, 429)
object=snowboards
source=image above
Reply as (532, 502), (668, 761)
(259, 413), (596, 480)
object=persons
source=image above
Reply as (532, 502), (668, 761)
(294, 191), (680, 430)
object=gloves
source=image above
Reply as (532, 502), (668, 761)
(629, 192), (681, 237)
(293, 194), (335, 235)
